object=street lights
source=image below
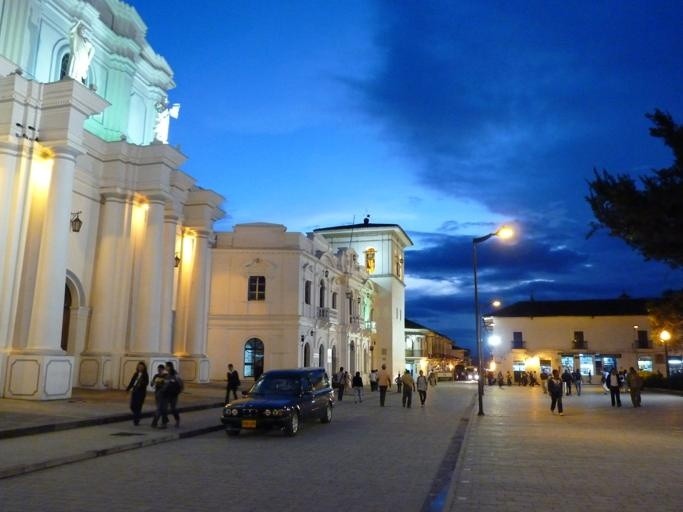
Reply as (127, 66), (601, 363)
(468, 222), (516, 417)
(479, 298), (502, 395)
(660, 327), (670, 376)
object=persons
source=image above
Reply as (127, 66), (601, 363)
(125, 362), (149, 426)
(484, 367), (643, 415)
(225, 364), (241, 405)
(154, 95), (181, 144)
(337, 364), (437, 408)
(657, 369), (681, 378)
(159, 361), (183, 430)
(151, 364), (170, 428)
(65, 20), (95, 84)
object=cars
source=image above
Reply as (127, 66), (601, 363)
(467, 372), (479, 381)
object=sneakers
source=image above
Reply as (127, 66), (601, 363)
(132, 415), (180, 429)
(549, 408), (565, 417)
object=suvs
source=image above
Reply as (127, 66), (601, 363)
(221, 366), (340, 436)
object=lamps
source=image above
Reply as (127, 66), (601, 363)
(322, 263), (329, 278)
(357, 295), (360, 304)
(70, 210), (83, 232)
(173, 251), (180, 268)
